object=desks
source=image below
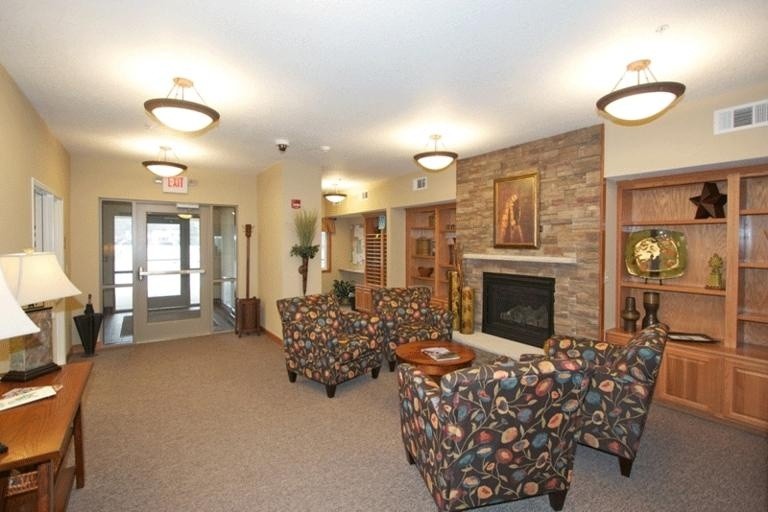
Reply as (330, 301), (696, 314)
(0, 362), (95, 511)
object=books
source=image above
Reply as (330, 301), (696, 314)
(0, 382), (64, 412)
(421, 346), (460, 362)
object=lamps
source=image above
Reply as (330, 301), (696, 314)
(0, 247), (83, 383)
(595, 56), (689, 123)
(325, 187), (348, 205)
(140, 75), (223, 136)
(415, 131), (460, 171)
(141, 141), (189, 180)
(0, 270), (43, 456)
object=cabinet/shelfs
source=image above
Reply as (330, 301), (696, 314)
(436, 207), (457, 302)
(406, 205), (435, 300)
(355, 211), (386, 313)
(720, 347), (767, 436)
(615, 179), (726, 347)
(604, 327), (725, 435)
(727, 165), (766, 354)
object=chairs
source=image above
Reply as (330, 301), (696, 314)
(275, 292), (387, 400)
(397, 355), (593, 510)
(371, 287), (453, 370)
(518, 321), (670, 476)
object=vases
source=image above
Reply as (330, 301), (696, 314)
(640, 291), (660, 332)
(447, 270), (461, 331)
(620, 295), (640, 333)
(461, 286), (474, 335)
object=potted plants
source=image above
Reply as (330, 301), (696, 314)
(332, 279), (351, 304)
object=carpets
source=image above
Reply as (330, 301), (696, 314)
(120, 307), (220, 338)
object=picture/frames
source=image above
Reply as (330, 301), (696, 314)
(494, 172), (538, 249)
